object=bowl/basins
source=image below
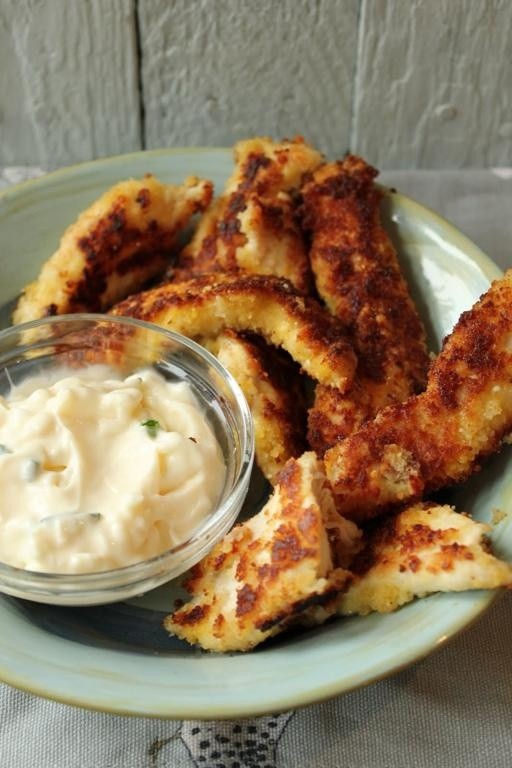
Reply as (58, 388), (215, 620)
(1, 143), (512, 722)
(0, 315), (260, 610)
